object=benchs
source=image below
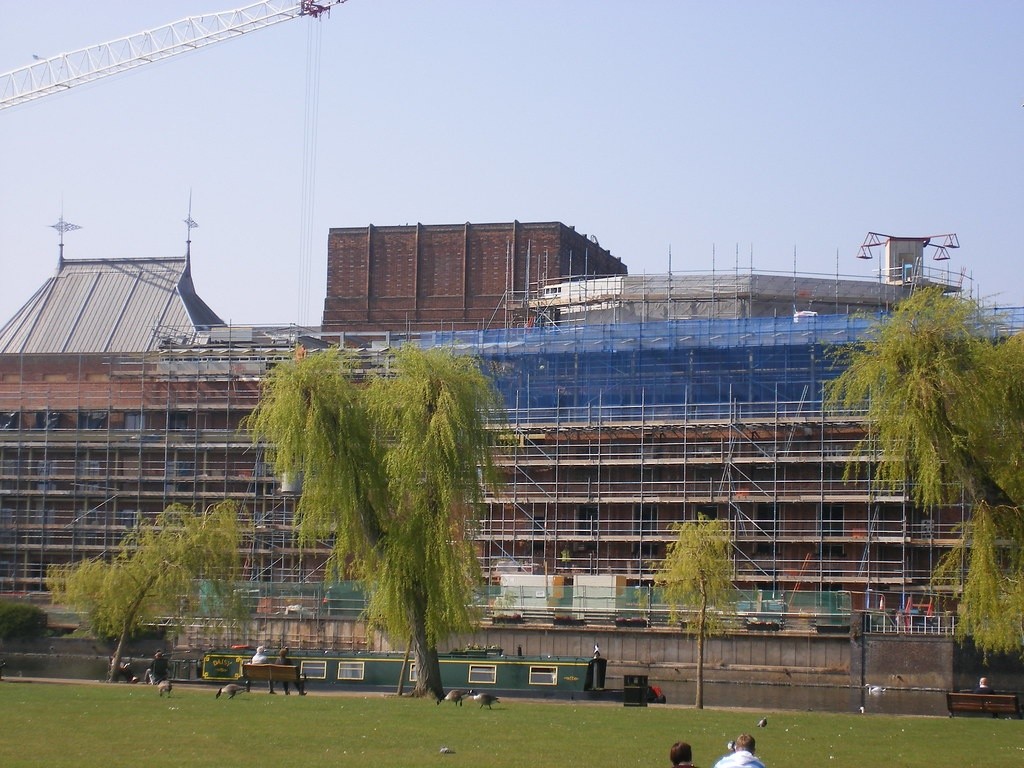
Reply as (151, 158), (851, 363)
(242, 661), (307, 696)
(946, 691), (1024, 720)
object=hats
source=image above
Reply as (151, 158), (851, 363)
(257, 646), (264, 654)
(155, 652), (162, 657)
(279, 648), (287, 656)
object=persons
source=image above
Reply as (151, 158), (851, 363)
(670, 742), (699, 768)
(756, 717), (767, 727)
(972, 677), (995, 695)
(110, 650), (138, 683)
(713, 734), (766, 768)
(275, 648), (307, 696)
(144, 652), (168, 685)
(251, 645), (276, 694)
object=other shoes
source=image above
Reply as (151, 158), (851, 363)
(269, 691), (275, 694)
(300, 692), (307, 695)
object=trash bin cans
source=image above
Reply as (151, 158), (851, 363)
(623, 674), (649, 707)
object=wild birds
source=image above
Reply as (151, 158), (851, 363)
(756, 717), (767, 728)
(466, 692), (501, 710)
(215, 684), (247, 700)
(158, 679), (173, 697)
(437, 689), (476, 709)
(727, 740), (736, 750)
(864, 683), (886, 694)
